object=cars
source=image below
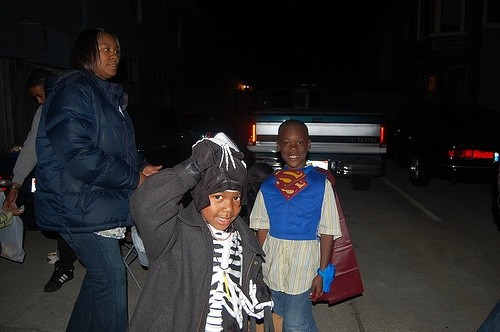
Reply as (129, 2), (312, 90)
(386, 95), (500, 189)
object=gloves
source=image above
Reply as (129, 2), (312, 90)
(189, 139), (222, 171)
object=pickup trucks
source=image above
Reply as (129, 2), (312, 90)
(239, 82), (388, 193)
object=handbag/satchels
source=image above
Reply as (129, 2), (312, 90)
(0, 190), (25, 263)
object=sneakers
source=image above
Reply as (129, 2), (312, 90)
(44, 261), (74, 293)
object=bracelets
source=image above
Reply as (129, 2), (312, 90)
(11, 185), (20, 190)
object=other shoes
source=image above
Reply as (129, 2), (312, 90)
(47, 252), (59, 264)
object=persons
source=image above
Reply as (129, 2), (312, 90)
(0, 206), (25, 228)
(128, 131), (274, 332)
(250, 119), (343, 332)
(2, 69), (77, 292)
(35, 26), (162, 332)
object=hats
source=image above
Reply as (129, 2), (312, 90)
(189, 147), (248, 211)
